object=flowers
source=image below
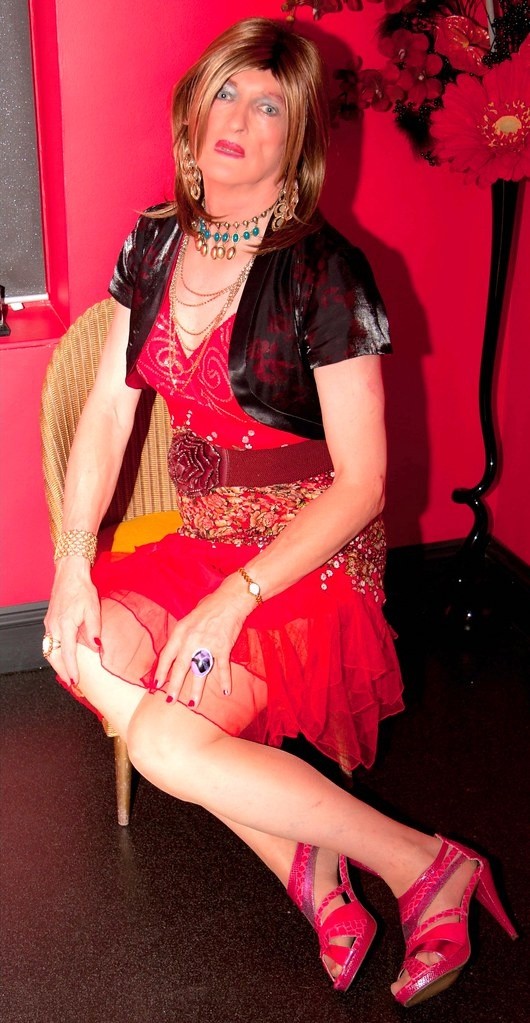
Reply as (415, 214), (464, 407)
(279, 0), (530, 187)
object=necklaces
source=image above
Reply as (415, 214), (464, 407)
(166, 193), (279, 393)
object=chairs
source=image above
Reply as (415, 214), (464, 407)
(41, 298), (183, 829)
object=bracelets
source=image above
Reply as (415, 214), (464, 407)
(53, 529), (99, 568)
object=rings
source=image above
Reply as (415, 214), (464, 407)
(41, 633), (61, 658)
(190, 647), (214, 677)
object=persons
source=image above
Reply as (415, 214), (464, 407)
(34, 19), (517, 1007)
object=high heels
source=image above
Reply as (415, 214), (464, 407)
(393, 833), (519, 1008)
(286, 841), (382, 993)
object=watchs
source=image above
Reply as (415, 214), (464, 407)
(238, 567), (264, 604)
